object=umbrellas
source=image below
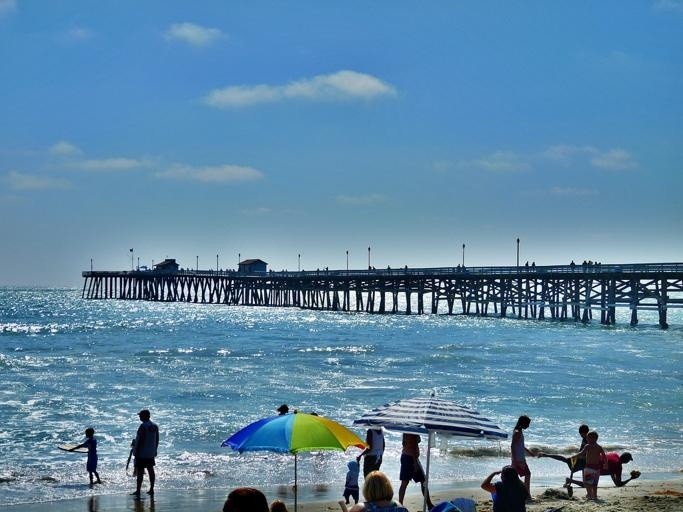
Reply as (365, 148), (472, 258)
(220, 409), (369, 512)
(354, 393), (508, 512)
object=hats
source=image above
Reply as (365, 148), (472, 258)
(622, 452), (633, 461)
(137, 410), (149, 415)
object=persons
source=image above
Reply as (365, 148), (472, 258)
(222, 486), (270, 511)
(129, 410), (159, 495)
(270, 498), (287, 511)
(537, 424), (641, 501)
(68, 428), (101, 485)
(399, 432), (435, 510)
(347, 470), (407, 511)
(509, 415), (536, 503)
(356, 426), (385, 479)
(480, 465), (526, 511)
